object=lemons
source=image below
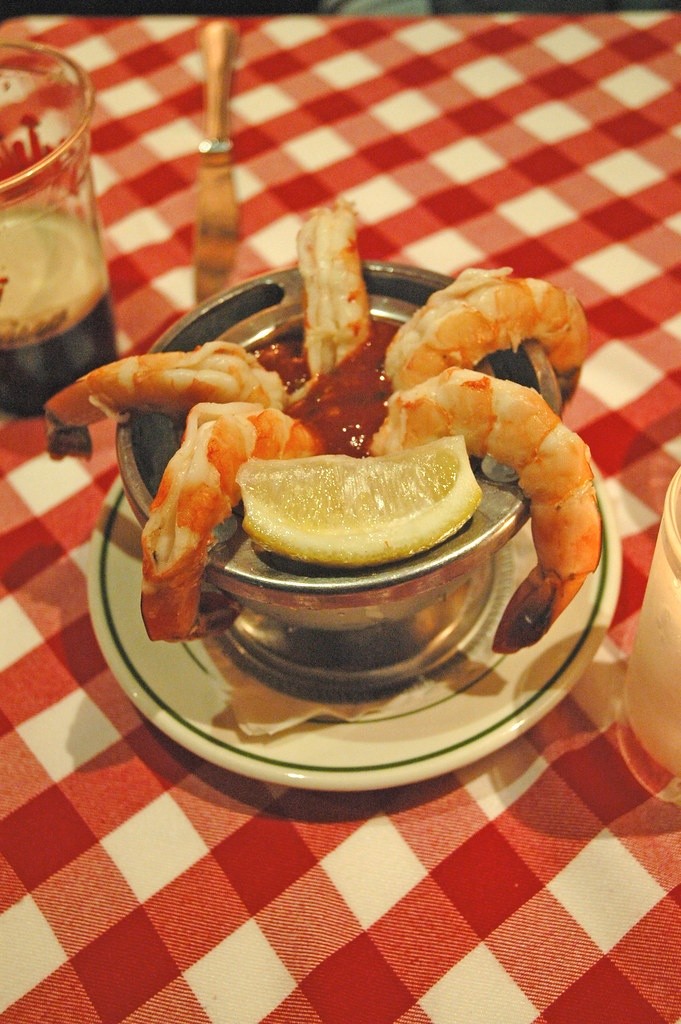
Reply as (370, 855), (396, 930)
(229, 434), (487, 568)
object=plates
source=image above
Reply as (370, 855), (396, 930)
(85, 459), (624, 791)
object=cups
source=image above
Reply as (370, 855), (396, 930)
(617, 463), (681, 802)
(0, 38), (122, 415)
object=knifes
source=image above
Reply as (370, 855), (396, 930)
(196, 19), (241, 305)
